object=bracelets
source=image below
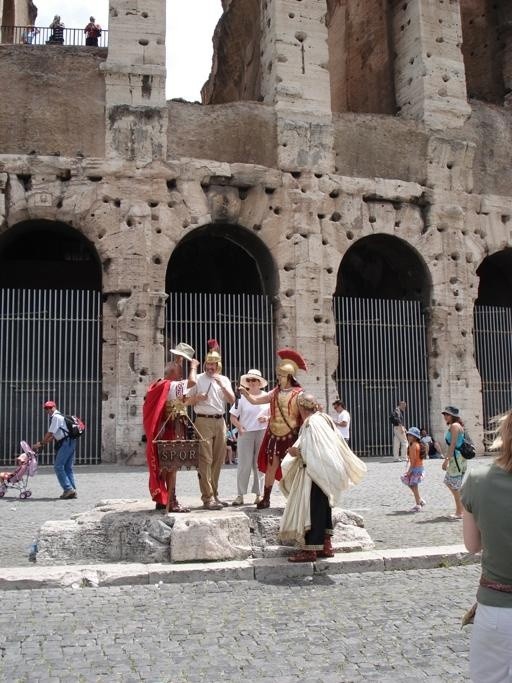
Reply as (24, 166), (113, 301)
(40, 441), (44, 446)
(445, 456), (450, 460)
(190, 368), (197, 371)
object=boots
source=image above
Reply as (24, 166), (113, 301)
(317, 536), (335, 557)
(257, 485), (272, 509)
(288, 550), (316, 562)
(167, 488), (192, 513)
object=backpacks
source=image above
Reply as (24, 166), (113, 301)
(419, 441), (429, 460)
(49, 413), (85, 442)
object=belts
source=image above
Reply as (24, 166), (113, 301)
(197, 414), (225, 419)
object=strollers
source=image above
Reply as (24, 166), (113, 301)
(0, 440), (39, 501)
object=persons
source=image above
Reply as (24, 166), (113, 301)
(441, 406), (467, 520)
(187, 351), (236, 510)
(400, 427), (426, 513)
(33, 401), (78, 499)
(420, 428), (445, 459)
(276, 392), (367, 562)
(0, 453), (27, 485)
(389, 401), (409, 462)
(229, 369), (270, 506)
(333, 400), (350, 445)
(237, 358), (306, 510)
(143, 354), (199, 513)
(457, 409), (512, 683)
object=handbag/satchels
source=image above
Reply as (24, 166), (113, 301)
(460, 442), (475, 460)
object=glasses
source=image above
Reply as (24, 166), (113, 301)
(245, 378), (257, 382)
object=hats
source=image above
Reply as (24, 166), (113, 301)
(168, 343), (195, 363)
(407, 427), (421, 439)
(239, 369), (267, 389)
(44, 401), (56, 408)
(441, 406), (461, 418)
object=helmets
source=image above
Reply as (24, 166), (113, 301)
(276, 361), (298, 390)
(202, 339), (223, 375)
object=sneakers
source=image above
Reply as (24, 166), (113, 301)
(254, 495), (261, 504)
(202, 496), (228, 511)
(60, 489), (77, 499)
(232, 495), (244, 505)
